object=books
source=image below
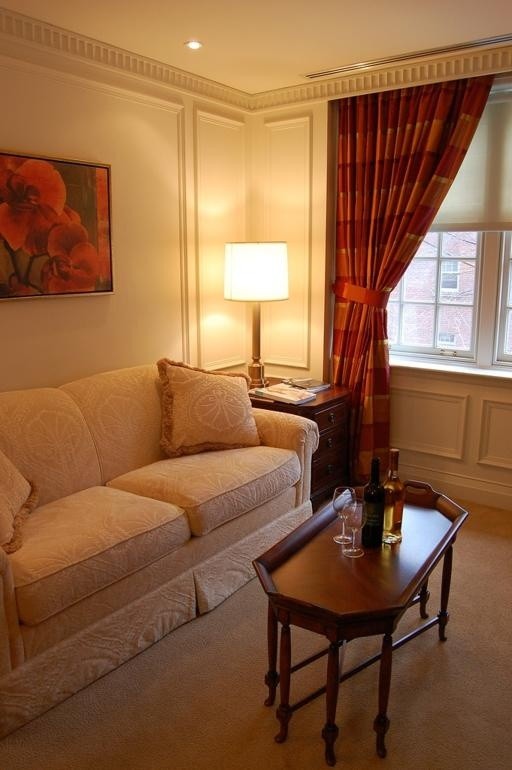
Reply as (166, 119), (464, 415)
(255, 384), (317, 407)
(287, 376), (333, 394)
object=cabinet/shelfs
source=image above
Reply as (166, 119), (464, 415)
(234, 376), (354, 513)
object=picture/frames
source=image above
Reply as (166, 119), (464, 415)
(0, 148), (115, 299)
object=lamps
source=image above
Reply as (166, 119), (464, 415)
(222, 239), (289, 385)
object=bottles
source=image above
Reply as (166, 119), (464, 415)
(363, 448), (404, 549)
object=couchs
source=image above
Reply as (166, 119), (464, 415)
(0, 360), (319, 739)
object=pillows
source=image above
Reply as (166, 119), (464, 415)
(155, 361), (261, 460)
(0, 447), (40, 555)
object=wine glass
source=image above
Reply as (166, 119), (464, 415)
(331, 485), (367, 557)
